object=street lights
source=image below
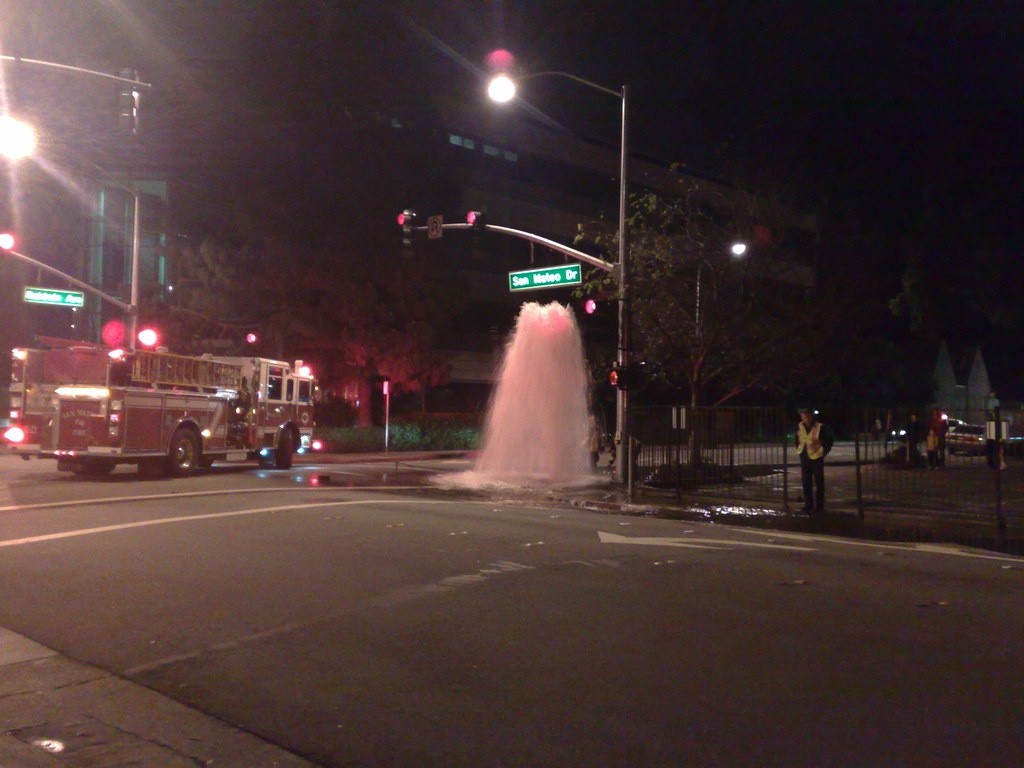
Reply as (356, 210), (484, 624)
(485, 70), (633, 480)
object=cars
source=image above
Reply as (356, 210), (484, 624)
(940, 419), (989, 456)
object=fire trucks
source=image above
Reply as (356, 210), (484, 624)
(5, 335), (321, 480)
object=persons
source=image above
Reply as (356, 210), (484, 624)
(905, 405), (949, 470)
(795, 407), (832, 510)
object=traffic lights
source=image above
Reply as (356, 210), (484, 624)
(0, 233), (16, 253)
(581, 295), (602, 318)
(244, 331), (261, 346)
(607, 369), (622, 388)
(396, 207), (417, 265)
(466, 210), (484, 230)
(136, 327), (160, 352)
